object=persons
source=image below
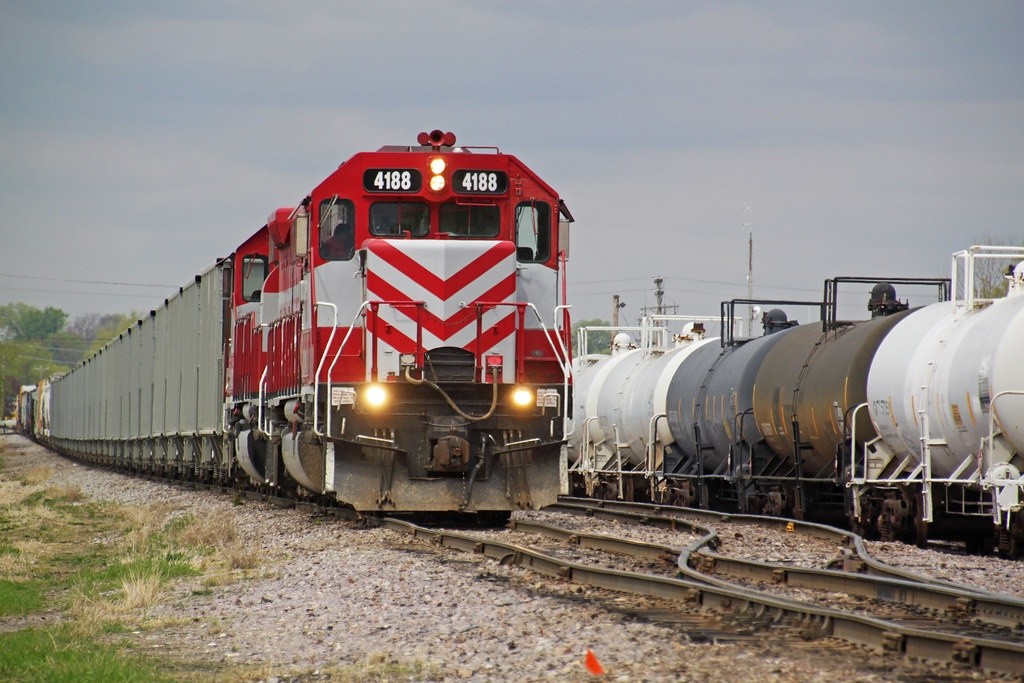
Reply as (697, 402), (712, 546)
(325, 224), (354, 259)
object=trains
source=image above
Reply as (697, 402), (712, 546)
(0, 128), (575, 513)
(562, 246), (1023, 561)
